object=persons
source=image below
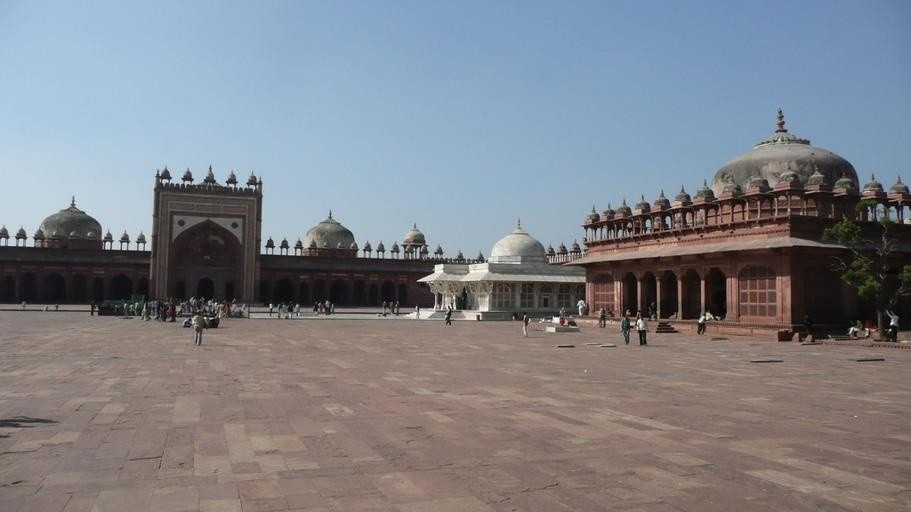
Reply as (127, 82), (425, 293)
(383, 299), (387, 317)
(192, 309), (206, 346)
(90, 293), (336, 328)
(390, 299), (394, 315)
(416, 303), (421, 320)
(557, 296), (722, 348)
(395, 300), (400, 316)
(847, 309), (901, 342)
(445, 305), (453, 326)
(522, 312), (530, 337)
